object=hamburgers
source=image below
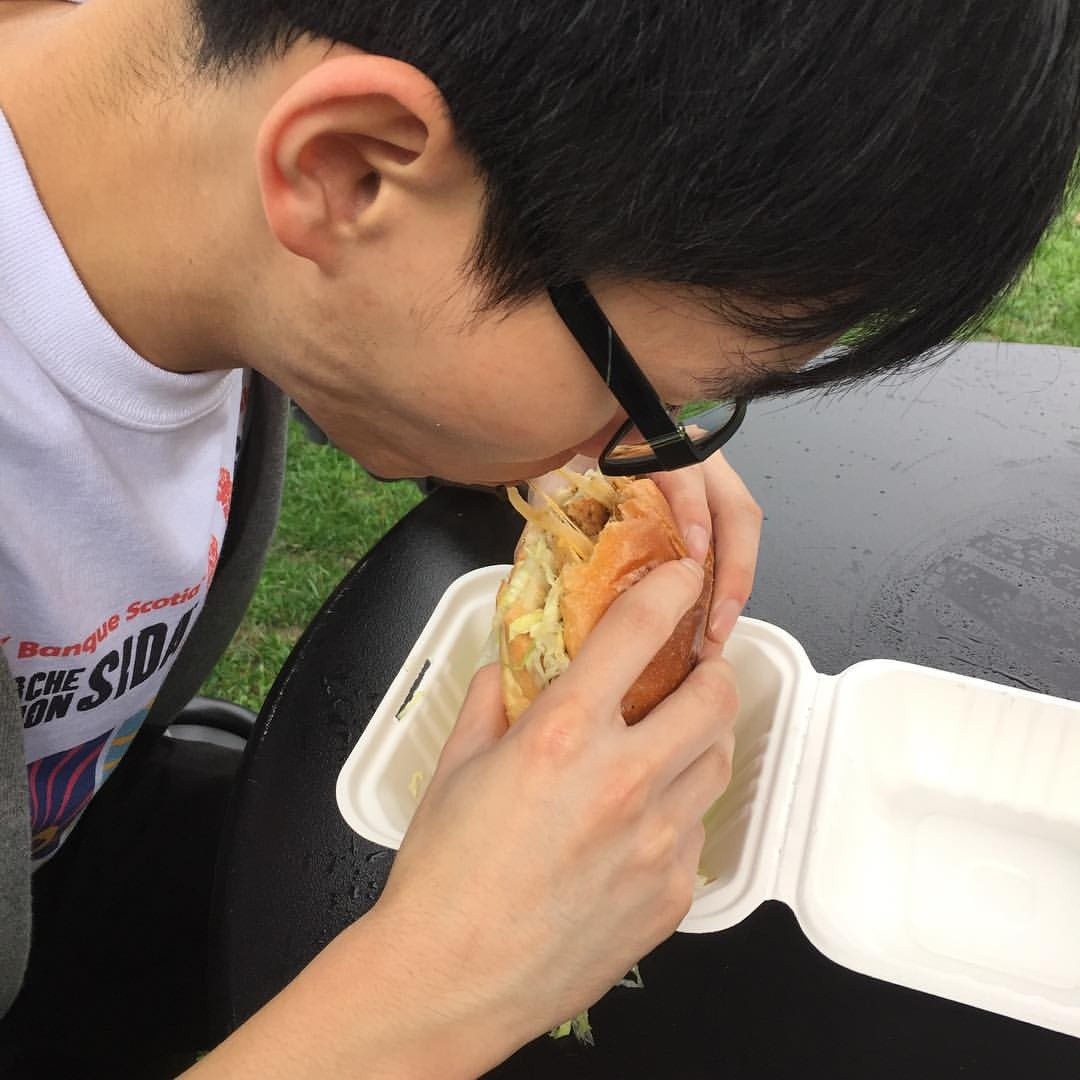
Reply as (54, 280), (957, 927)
(492, 468), (718, 731)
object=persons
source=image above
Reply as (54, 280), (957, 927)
(0, 0), (1080, 1080)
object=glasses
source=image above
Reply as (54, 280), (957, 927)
(449, 96), (748, 478)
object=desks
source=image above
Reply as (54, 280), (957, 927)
(218, 341), (1080, 1080)
